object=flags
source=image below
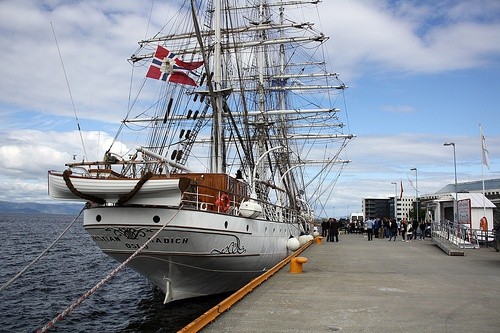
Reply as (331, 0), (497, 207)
(481, 133), (490, 170)
(145, 46), (203, 86)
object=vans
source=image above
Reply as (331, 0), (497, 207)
(349, 212), (365, 234)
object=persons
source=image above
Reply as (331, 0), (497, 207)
(321, 218), (339, 242)
(346, 220), (364, 234)
(365, 217), (431, 241)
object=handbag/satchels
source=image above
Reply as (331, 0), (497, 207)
(408, 225), (414, 232)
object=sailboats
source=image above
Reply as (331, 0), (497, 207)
(47, 0), (357, 308)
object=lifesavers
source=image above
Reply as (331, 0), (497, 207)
(220, 193), (231, 213)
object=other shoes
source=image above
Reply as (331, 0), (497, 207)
(419, 239), (421, 240)
(406, 240), (409, 242)
(402, 240), (405, 241)
(422, 239), (424, 241)
(410, 241), (411, 242)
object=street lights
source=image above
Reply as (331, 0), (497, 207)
(443, 142), (460, 235)
(410, 168), (418, 222)
(391, 182), (397, 221)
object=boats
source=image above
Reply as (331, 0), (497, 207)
(48, 170), (192, 200)
